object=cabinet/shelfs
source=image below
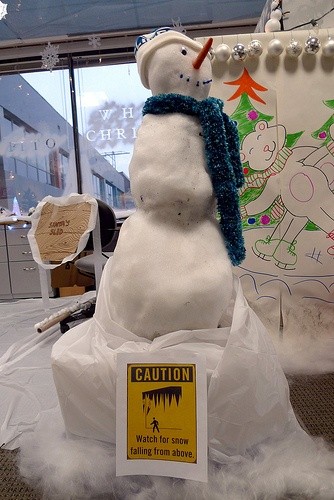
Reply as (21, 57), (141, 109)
(0, 225), (56, 300)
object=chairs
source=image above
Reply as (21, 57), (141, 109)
(60, 199), (117, 333)
